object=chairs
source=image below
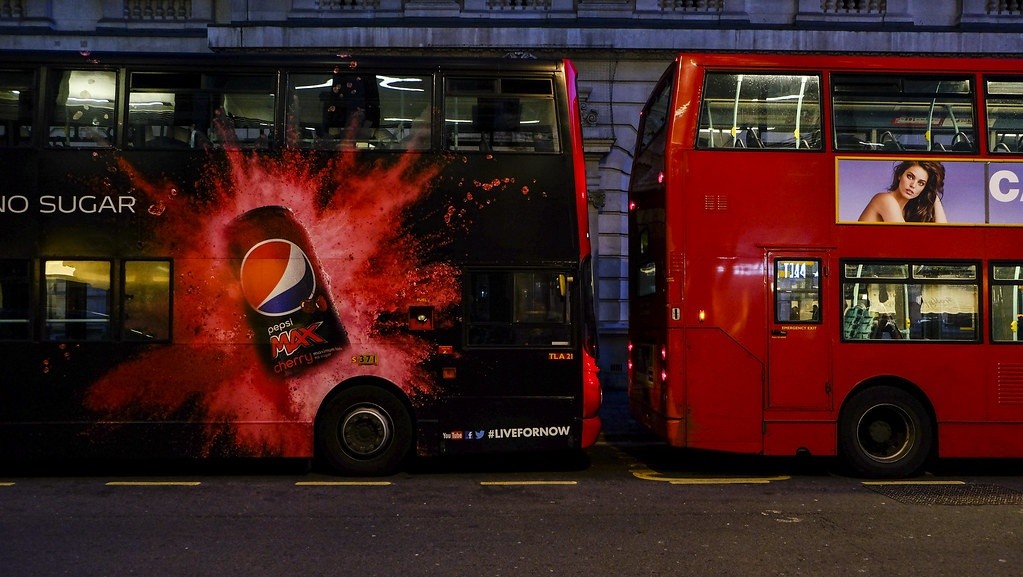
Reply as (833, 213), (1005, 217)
(853, 310), (885, 338)
(1017, 136), (1023, 152)
(844, 305), (868, 339)
(799, 139), (811, 149)
(734, 138), (745, 147)
(950, 131), (974, 151)
(746, 128), (765, 148)
(933, 143), (948, 150)
(880, 131), (906, 151)
(992, 142), (1010, 152)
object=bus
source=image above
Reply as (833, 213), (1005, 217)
(616, 52), (1023, 477)
(1, 43), (602, 479)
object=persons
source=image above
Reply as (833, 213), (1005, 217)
(791, 305), (799, 321)
(812, 305), (818, 320)
(858, 301), (866, 310)
(856, 160), (947, 224)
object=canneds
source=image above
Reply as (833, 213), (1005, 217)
(222, 206), (350, 382)
